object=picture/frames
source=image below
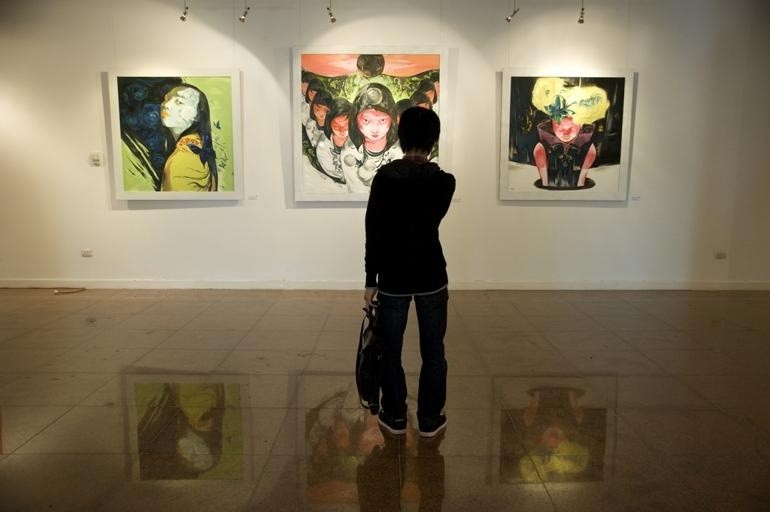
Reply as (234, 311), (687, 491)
(499, 68), (634, 201)
(490, 374), (617, 493)
(296, 372), (447, 512)
(292, 44), (449, 202)
(108, 68), (245, 202)
(125, 373), (254, 489)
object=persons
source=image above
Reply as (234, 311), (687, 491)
(522, 388), (585, 451)
(167, 383), (227, 475)
(298, 374), (423, 503)
(156, 82), (218, 193)
(532, 116), (599, 189)
(293, 73), (439, 189)
(362, 106), (457, 439)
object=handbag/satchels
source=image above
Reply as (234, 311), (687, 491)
(356, 303), (383, 415)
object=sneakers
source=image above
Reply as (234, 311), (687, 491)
(378, 409), (408, 436)
(418, 408), (448, 438)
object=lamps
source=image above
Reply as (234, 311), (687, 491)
(180, 0), (188, 21)
(505, 0), (520, 23)
(239, 0), (250, 22)
(327, 0), (336, 23)
(578, 0), (585, 23)
